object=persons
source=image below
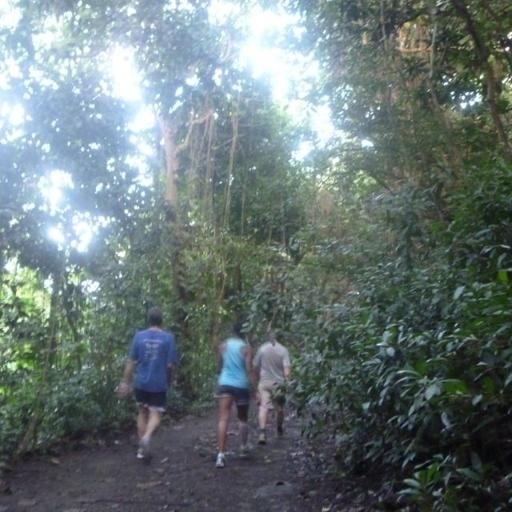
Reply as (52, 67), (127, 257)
(117, 307), (177, 463)
(212, 323), (256, 468)
(255, 328), (290, 444)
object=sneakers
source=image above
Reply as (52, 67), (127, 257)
(215, 455), (225, 468)
(257, 434), (266, 444)
(239, 446), (251, 458)
(136, 439), (152, 461)
(276, 431), (282, 438)
